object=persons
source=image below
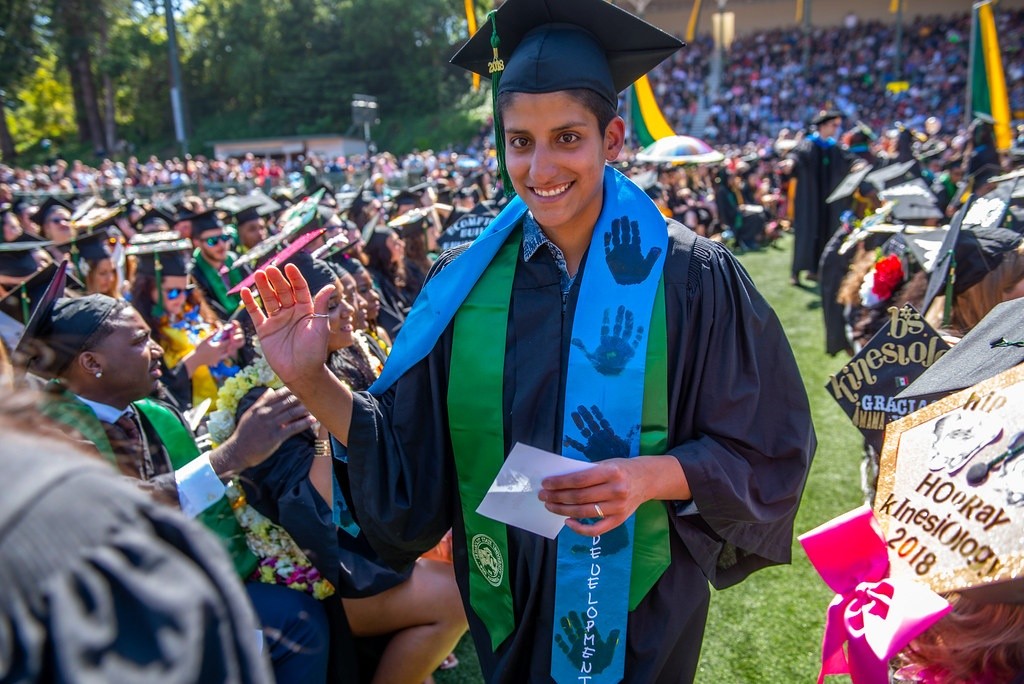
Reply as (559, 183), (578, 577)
(240, 0), (818, 684)
(0, 136), (789, 684)
(777, 107), (1024, 684)
(469, 0), (1024, 141)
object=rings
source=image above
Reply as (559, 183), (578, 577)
(595, 504), (604, 518)
(312, 313), (329, 318)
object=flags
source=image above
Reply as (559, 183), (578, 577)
(964, 1), (1012, 152)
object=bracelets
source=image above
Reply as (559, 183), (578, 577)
(313, 439), (332, 457)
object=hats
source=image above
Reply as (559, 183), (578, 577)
(809, 109), (1024, 316)
(448, 0), (687, 198)
(0, 182), (453, 382)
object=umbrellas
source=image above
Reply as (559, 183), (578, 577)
(636, 135), (724, 166)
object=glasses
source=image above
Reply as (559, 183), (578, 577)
(0, 282), (14, 292)
(162, 285), (198, 299)
(48, 218), (71, 222)
(199, 233), (231, 246)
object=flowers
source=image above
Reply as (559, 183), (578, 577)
(208, 344), (333, 600)
